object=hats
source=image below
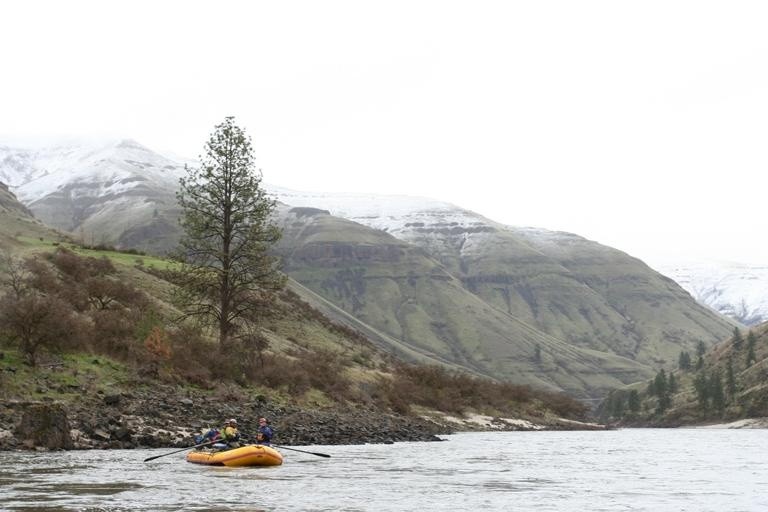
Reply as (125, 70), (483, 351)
(224, 419), (237, 424)
(260, 418), (266, 422)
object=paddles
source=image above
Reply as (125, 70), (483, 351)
(144, 438), (225, 462)
(270, 443), (332, 459)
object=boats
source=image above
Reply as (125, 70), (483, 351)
(186, 445), (283, 467)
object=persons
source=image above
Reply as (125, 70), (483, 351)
(254, 417), (273, 447)
(220, 418), (231, 442)
(224, 418), (241, 448)
